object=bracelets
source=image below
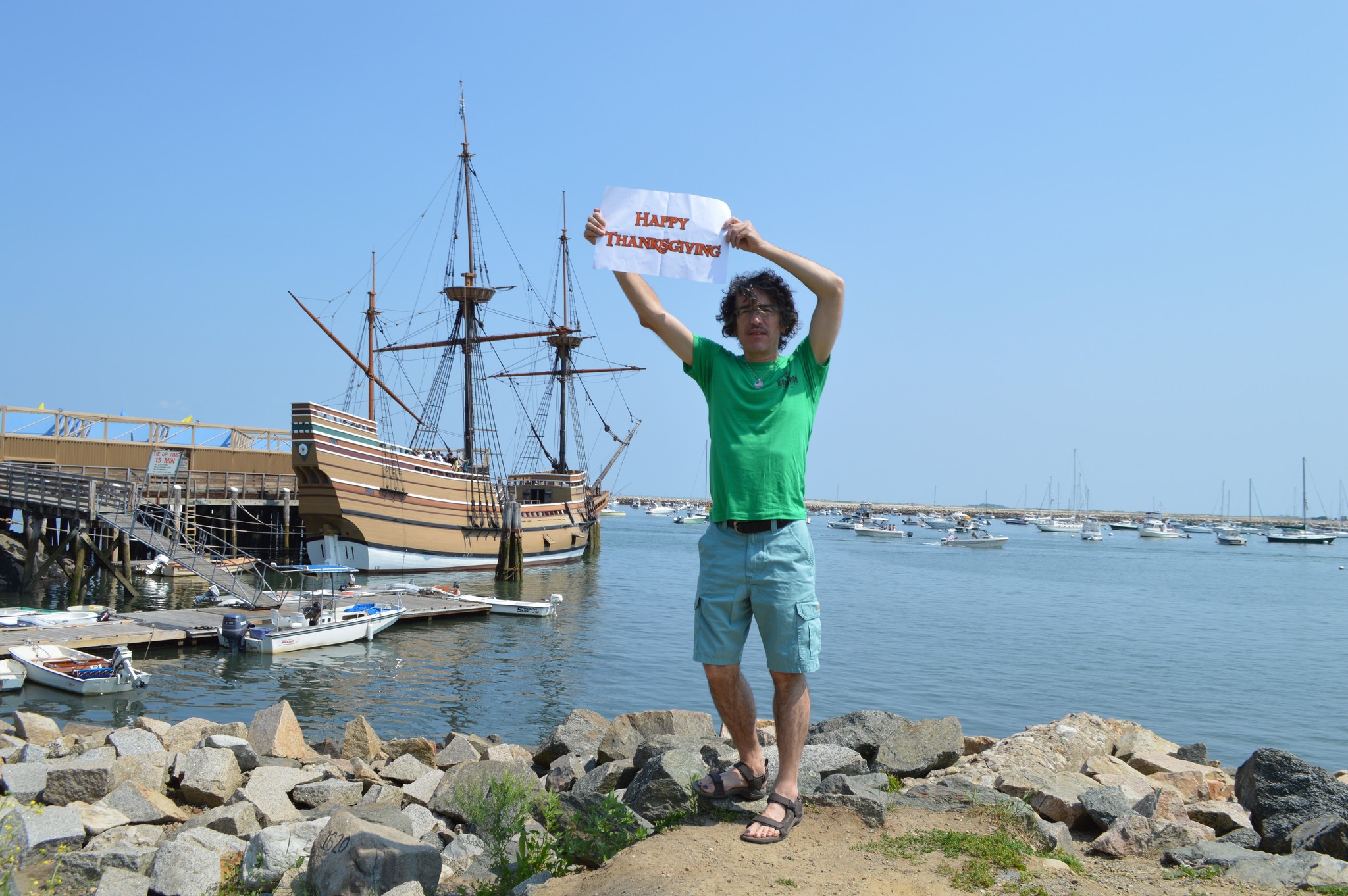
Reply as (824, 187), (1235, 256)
(460, 458), (461, 459)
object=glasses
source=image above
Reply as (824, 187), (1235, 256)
(734, 304), (781, 320)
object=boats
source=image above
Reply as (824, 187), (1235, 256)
(903, 512), (1010, 548)
(608, 498), (621, 506)
(600, 507), (626, 517)
(674, 512), (707, 524)
(883, 508), (902, 516)
(145, 553), (262, 577)
(1109, 495), (1213, 538)
(805, 508), (832, 516)
(418, 582), (563, 617)
(842, 501), (888, 526)
(627, 500), (712, 515)
(0, 604), (118, 630)
(1216, 528), (1248, 546)
(0, 639), (152, 696)
(1079, 516), (1104, 541)
(827, 518), (913, 538)
(805, 518), (812, 524)
(193, 563), (419, 654)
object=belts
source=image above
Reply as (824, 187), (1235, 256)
(717, 518), (791, 534)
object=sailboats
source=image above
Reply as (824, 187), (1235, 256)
(287, 71), (649, 577)
(1003, 447), (1106, 532)
(831, 484), (842, 514)
(975, 490), (995, 519)
(686, 440), (710, 520)
(933, 486), (941, 519)
(1211, 457), (1348, 544)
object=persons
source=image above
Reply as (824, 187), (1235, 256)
(892, 524), (897, 531)
(971, 532), (978, 538)
(405, 450), (469, 478)
(847, 520), (849, 523)
(907, 516), (911, 521)
(584, 207), (845, 845)
(888, 523), (891, 531)
(882, 523), (887, 530)
(921, 517), (924, 520)
(955, 537), (959, 541)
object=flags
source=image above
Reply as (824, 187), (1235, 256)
(946, 533), (955, 541)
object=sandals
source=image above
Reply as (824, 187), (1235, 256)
(692, 758), (769, 799)
(741, 793), (804, 843)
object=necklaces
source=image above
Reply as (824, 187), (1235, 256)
(741, 354), (775, 388)
